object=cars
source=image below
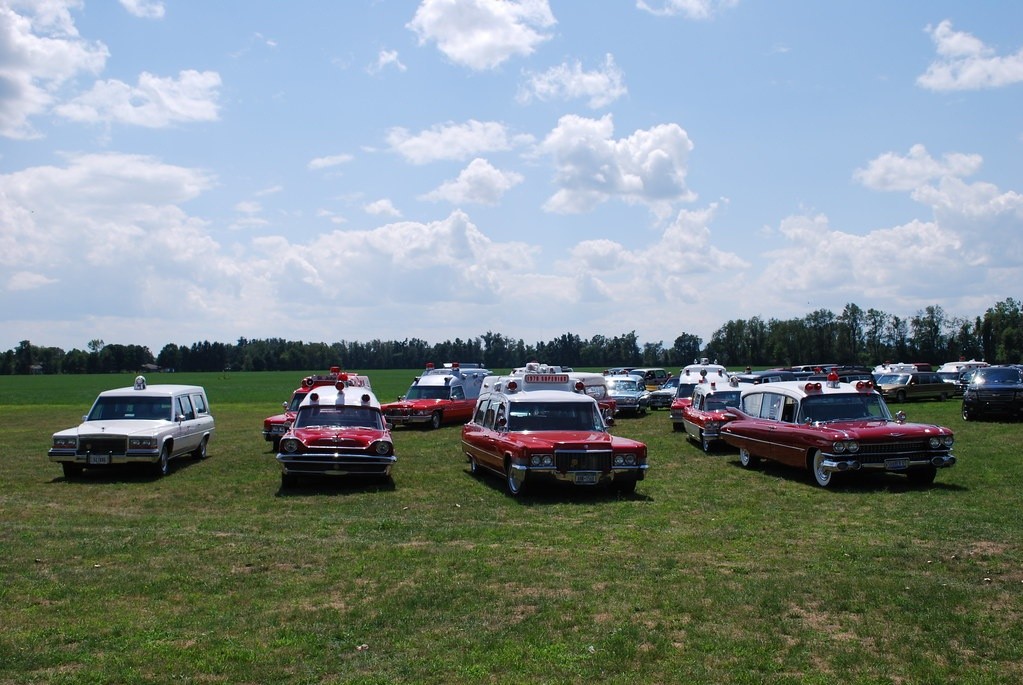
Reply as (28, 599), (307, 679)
(716, 370), (958, 492)
(670, 357), (725, 431)
(46, 376), (215, 478)
(459, 374), (650, 499)
(377, 361), (496, 429)
(262, 365), (371, 454)
(874, 371), (956, 405)
(681, 376), (760, 454)
(479, 362), (679, 417)
(724, 359), (991, 401)
(962, 366), (1023, 420)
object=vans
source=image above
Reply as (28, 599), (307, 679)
(277, 382), (398, 481)
